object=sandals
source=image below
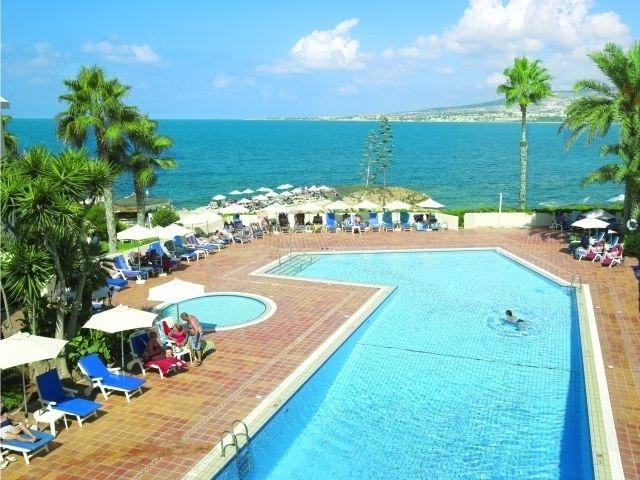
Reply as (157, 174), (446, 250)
(195, 361), (202, 367)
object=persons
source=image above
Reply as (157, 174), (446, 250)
(600, 246), (619, 263)
(342, 212), (350, 225)
(416, 215), (437, 223)
(586, 240), (604, 254)
(0, 411), (42, 444)
(295, 212), (303, 226)
(505, 309), (536, 334)
(145, 331), (174, 362)
(279, 212), (289, 231)
(313, 212), (321, 231)
(355, 214), (362, 226)
(168, 323), (188, 346)
(261, 216), (271, 236)
(179, 312), (203, 368)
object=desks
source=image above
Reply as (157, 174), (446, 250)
(33, 405), (71, 439)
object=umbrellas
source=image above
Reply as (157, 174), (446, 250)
(382, 200), (413, 223)
(354, 199), (381, 220)
(79, 303), (159, 372)
(327, 199), (352, 223)
(148, 277), (207, 322)
(570, 216), (610, 240)
(115, 200), (250, 272)
(0, 328), (71, 430)
(608, 193), (628, 206)
(209, 183), (332, 206)
(415, 196), (442, 219)
(259, 200), (290, 223)
(296, 202), (324, 224)
(579, 207), (617, 236)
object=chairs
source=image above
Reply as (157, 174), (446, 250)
(1, 421), (57, 467)
(551, 208), (629, 269)
(77, 351), (148, 403)
(33, 366), (104, 427)
(155, 316), (216, 365)
(125, 329), (186, 380)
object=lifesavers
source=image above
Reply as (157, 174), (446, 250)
(626, 219), (639, 231)
